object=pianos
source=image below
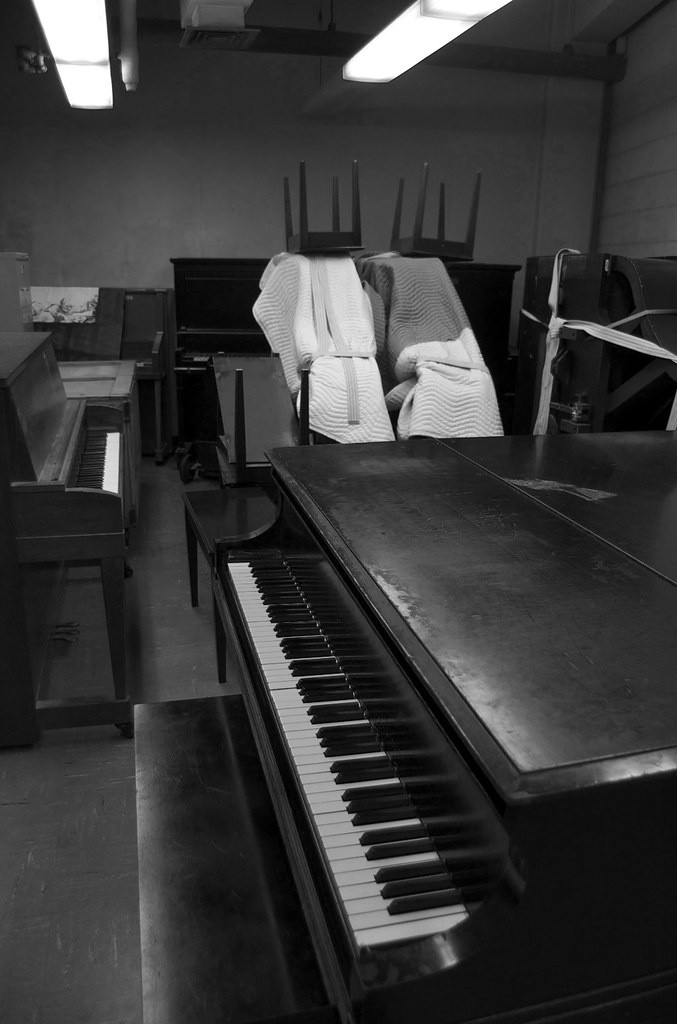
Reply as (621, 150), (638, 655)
(167, 256), (523, 465)
(0, 245), (148, 747)
(179, 437), (677, 1024)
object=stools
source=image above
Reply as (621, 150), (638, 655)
(217, 370), (309, 484)
(180, 488), (277, 684)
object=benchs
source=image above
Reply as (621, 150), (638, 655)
(133, 693), (343, 1024)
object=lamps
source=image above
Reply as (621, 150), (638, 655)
(297, 0), (513, 119)
(32, 0), (114, 110)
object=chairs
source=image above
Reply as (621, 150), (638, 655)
(389, 163), (481, 260)
(283, 160), (366, 253)
